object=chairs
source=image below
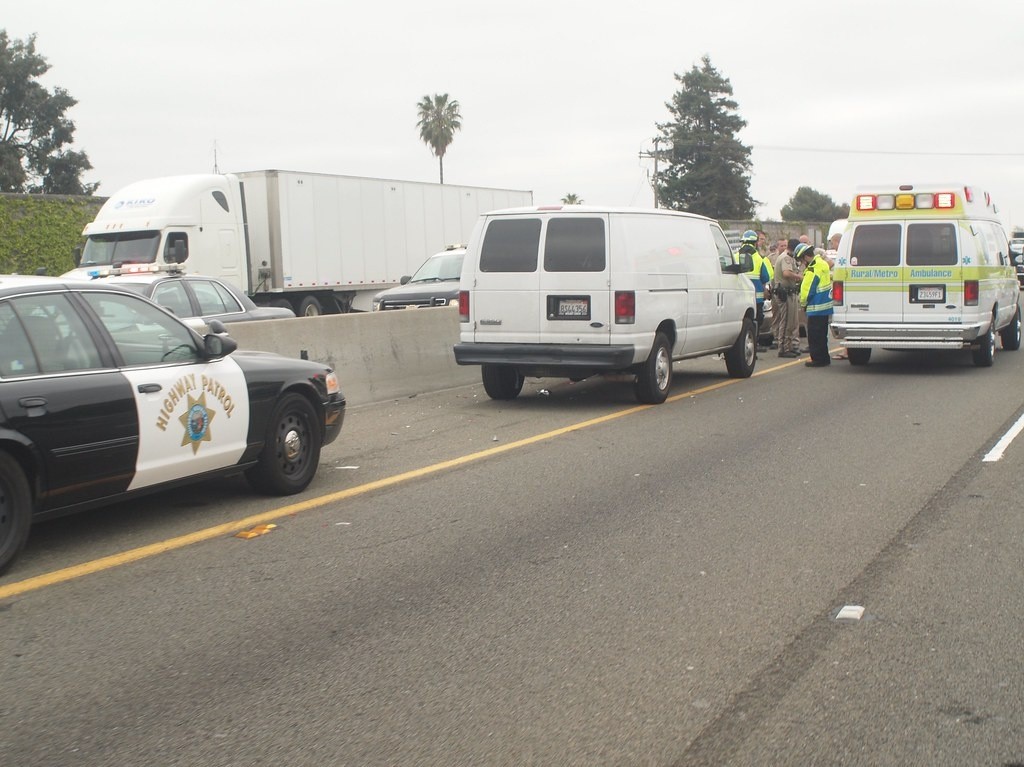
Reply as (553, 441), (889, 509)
(158, 292), (184, 311)
(0, 316), (76, 377)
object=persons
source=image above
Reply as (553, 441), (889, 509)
(766, 245), (779, 260)
(768, 238), (789, 349)
(832, 234), (849, 359)
(731, 230), (774, 359)
(793, 243), (834, 368)
(797, 235), (835, 353)
(752, 228), (769, 353)
(772, 238), (804, 358)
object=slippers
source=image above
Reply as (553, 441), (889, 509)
(832, 355), (848, 360)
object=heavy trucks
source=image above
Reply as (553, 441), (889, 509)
(60, 168), (534, 318)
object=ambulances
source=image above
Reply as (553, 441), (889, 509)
(827, 180), (1022, 367)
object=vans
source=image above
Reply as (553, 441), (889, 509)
(453, 203), (761, 405)
(1007, 232), (1024, 289)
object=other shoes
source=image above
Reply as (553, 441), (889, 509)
(757, 343), (830, 367)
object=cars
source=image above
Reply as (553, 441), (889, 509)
(0, 273), (347, 577)
(34, 262), (298, 364)
(372, 243), (467, 315)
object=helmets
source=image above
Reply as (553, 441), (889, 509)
(739, 230), (758, 242)
(794, 243), (815, 260)
